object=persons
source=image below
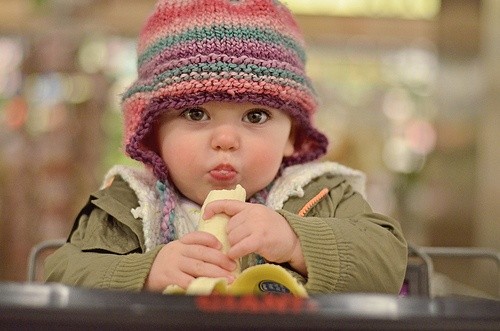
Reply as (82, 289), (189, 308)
(42, 0), (409, 294)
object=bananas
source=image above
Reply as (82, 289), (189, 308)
(195, 184), (247, 279)
